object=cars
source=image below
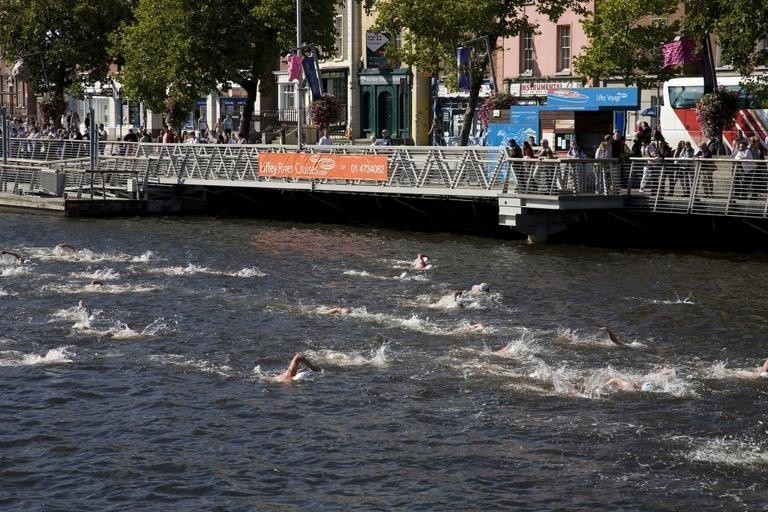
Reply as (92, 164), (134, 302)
(444, 136), (480, 145)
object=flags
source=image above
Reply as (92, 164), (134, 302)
(659, 36), (704, 68)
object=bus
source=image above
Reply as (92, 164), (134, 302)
(659, 76), (768, 158)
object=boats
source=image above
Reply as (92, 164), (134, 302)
(60, 190), (151, 218)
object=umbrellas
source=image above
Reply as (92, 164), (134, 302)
(640, 107), (660, 122)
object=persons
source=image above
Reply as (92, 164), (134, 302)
(369, 135), (379, 151)
(48, 251), (153, 340)
(376, 129), (394, 146)
(504, 120), (767, 193)
(1, 110), (247, 158)
(319, 128), (333, 146)
(427, 117), (443, 146)
(277, 251), (768, 392)
